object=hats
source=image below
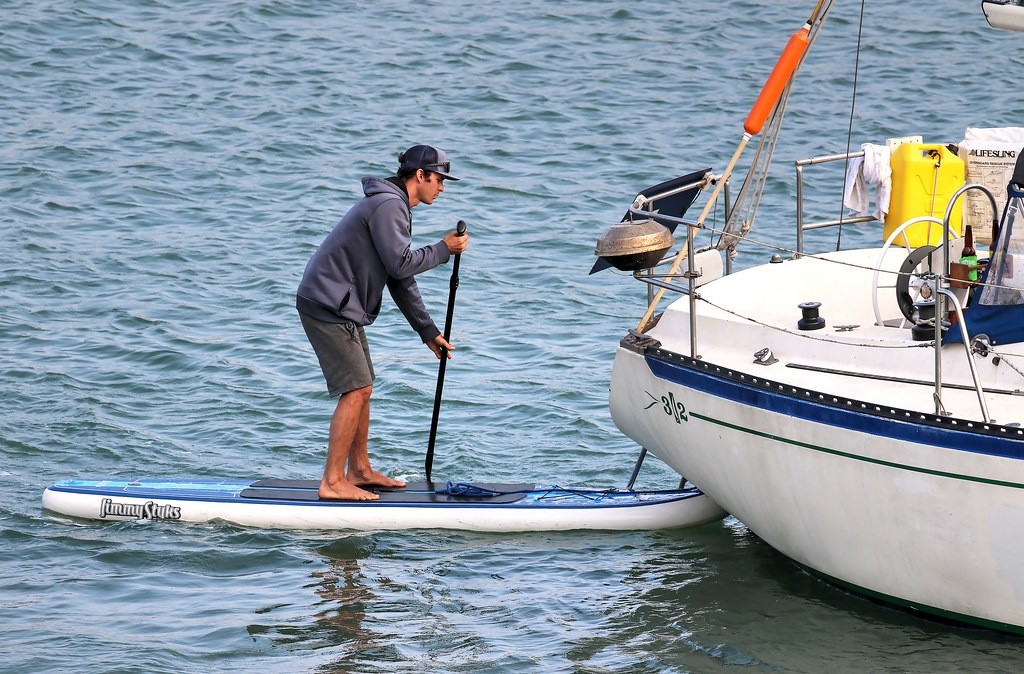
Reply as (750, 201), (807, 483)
(400, 145), (460, 180)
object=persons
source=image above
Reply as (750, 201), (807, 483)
(295, 145), (468, 500)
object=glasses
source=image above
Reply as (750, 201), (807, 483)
(421, 161), (451, 172)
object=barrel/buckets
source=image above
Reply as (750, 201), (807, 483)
(884, 141), (966, 248)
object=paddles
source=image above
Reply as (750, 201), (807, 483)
(421, 219), (467, 483)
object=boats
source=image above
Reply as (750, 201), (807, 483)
(974, 0), (1023, 30)
(587, 0), (1023, 642)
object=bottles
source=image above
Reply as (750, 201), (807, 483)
(961, 225), (978, 281)
(989, 219), (1000, 259)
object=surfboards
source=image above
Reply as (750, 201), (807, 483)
(37, 473), (731, 536)
